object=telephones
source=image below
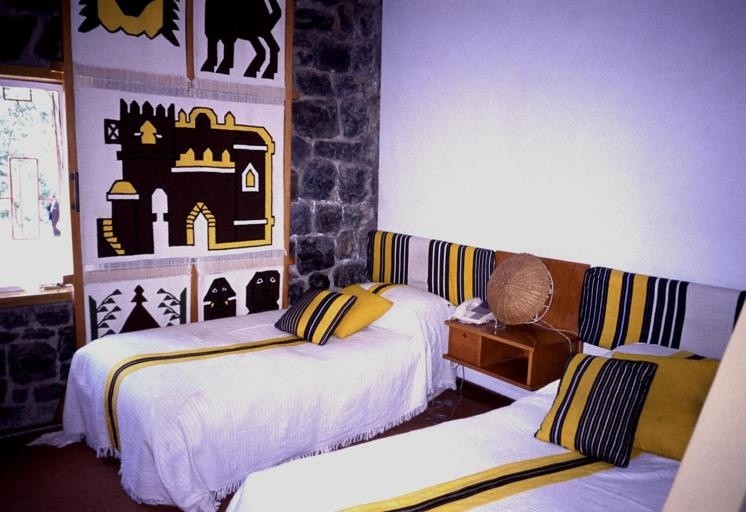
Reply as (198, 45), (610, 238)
(454, 297), (493, 325)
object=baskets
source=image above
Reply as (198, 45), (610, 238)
(487, 253), (554, 325)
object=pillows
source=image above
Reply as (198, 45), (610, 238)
(274, 285), (356, 347)
(606, 352), (721, 463)
(330, 281), (392, 338)
(534, 352), (660, 469)
(348, 282), (456, 337)
(583, 342), (725, 358)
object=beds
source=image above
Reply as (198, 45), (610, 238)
(57, 280), (455, 512)
(225, 340), (721, 509)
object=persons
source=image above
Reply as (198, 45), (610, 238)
(43, 195), (61, 238)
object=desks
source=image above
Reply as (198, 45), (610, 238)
(442, 317), (579, 396)
